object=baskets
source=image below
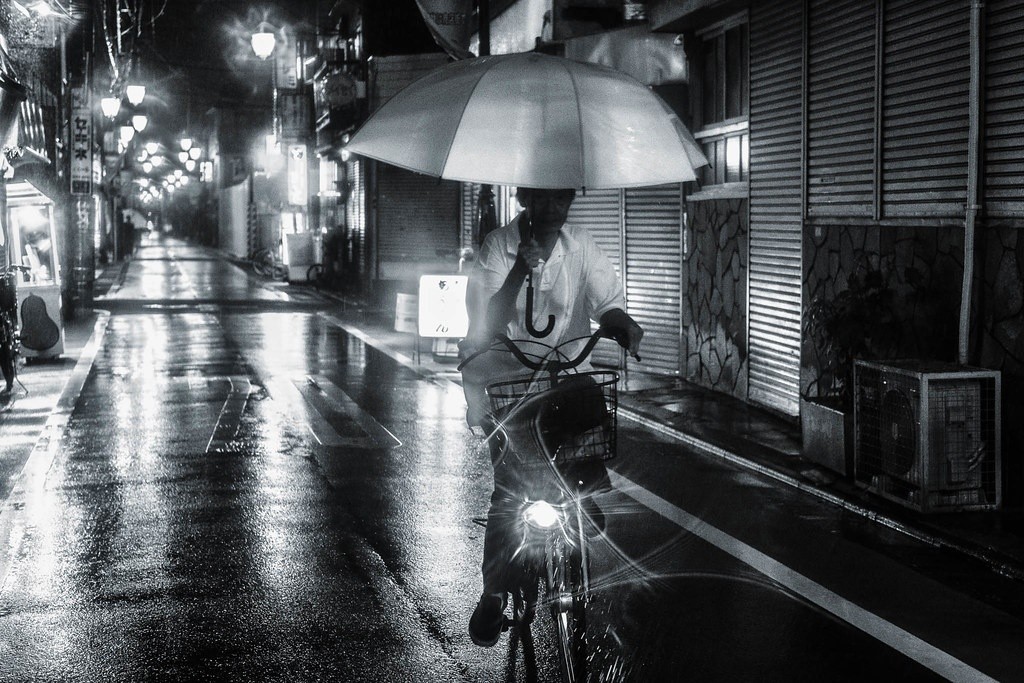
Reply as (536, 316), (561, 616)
(488, 372), (620, 472)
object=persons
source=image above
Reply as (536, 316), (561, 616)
(458, 187), (643, 648)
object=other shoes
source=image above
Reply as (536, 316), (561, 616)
(468, 595), (508, 646)
(572, 498), (606, 542)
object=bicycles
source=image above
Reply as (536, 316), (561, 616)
(454, 326), (641, 683)
(0, 264), (31, 396)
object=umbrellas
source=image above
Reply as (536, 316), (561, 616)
(347, 36), (715, 340)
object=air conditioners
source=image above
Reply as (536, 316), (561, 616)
(878, 372), (982, 491)
